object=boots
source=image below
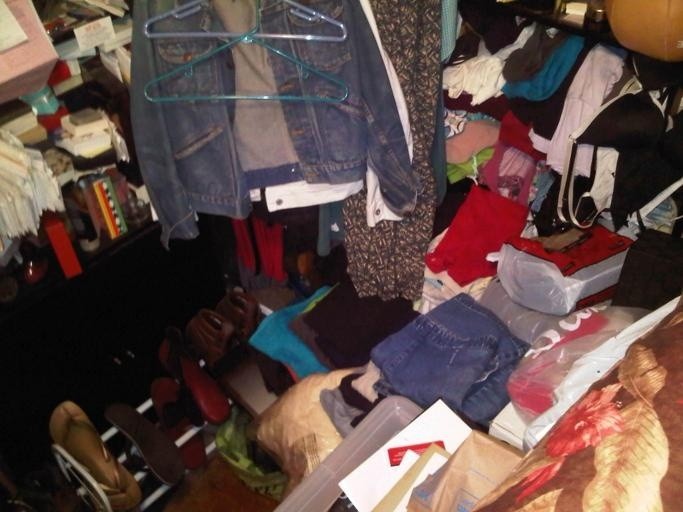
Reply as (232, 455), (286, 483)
(184, 309), (235, 368)
(215, 286), (258, 355)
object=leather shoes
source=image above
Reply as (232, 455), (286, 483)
(159, 337), (231, 425)
(149, 377), (185, 414)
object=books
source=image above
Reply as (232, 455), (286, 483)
(1, 98), (49, 145)
(75, 0), (135, 55)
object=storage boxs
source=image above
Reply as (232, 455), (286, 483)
(269, 394), (428, 512)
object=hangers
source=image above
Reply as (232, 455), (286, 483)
(144, 0), (349, 107)
(142, 0), (348, 43)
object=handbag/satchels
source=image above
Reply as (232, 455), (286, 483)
(215, 403), (287, 500)
(556, 84), (683, 229)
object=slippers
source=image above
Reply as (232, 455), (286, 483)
(49, 400), (127, 498)
(101, 461), (142, 512)
(152, 378), (208, 471)
(104, 403), (187, 487)
